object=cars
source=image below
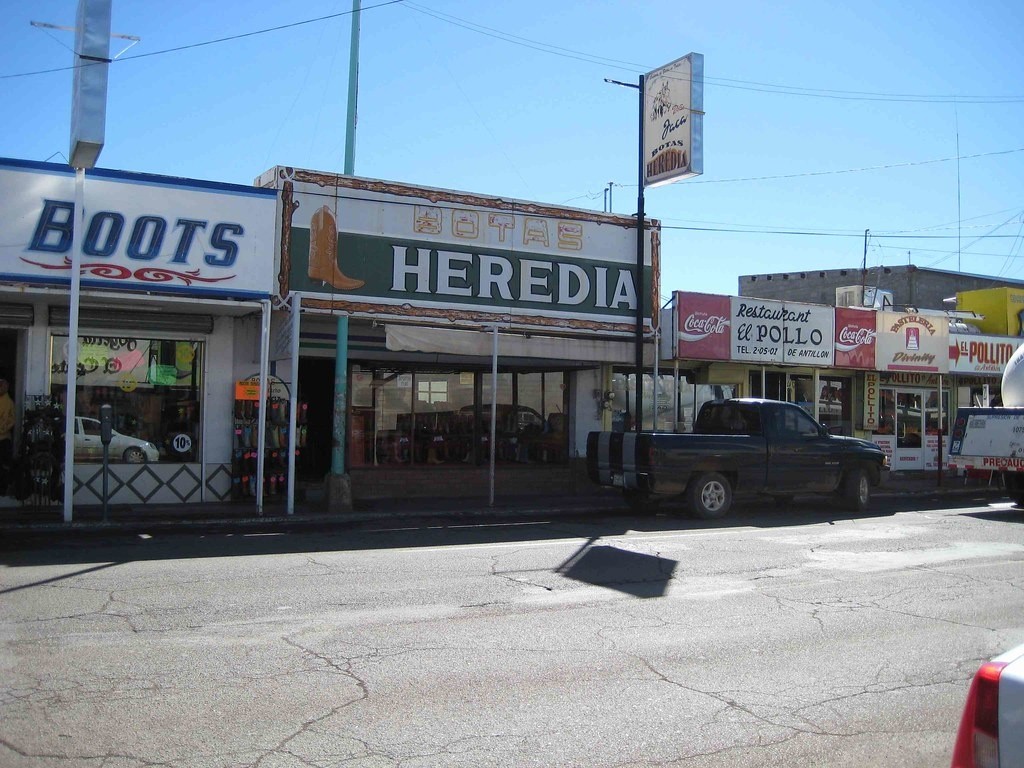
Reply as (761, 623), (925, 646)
(952, 639), (1024, 768)
(51, 415), (161, 462)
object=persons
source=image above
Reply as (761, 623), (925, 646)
(0, 377), (14, 496)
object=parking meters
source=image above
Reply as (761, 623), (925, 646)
(99, 403), (114, 525)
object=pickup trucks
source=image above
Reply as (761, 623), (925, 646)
(585, 397), (886, 520)
(378, 403), (552, 463)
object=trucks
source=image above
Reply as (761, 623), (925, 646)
(945, 404), (1024, 510)
